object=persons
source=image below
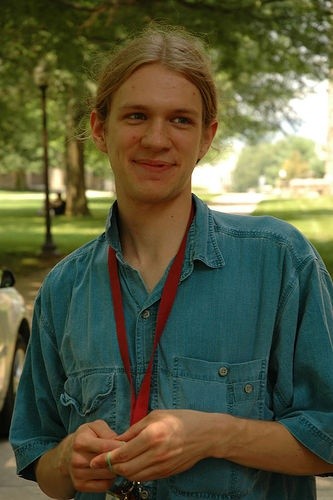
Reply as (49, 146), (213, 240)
(10, 24), (333, 500)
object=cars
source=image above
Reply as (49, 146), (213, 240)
(0, 268), (34, 439)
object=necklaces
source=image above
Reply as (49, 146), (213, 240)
(108, 197), (199, 499)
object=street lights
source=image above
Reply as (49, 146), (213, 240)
(34, 58), (59, 261)
